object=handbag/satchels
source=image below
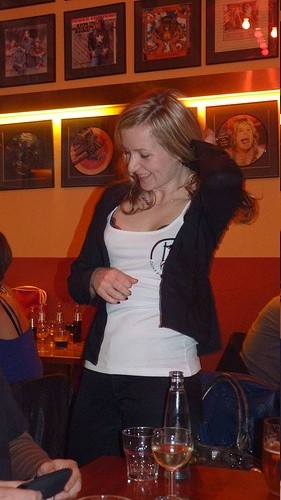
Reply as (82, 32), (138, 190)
(189, 369), (256, 472)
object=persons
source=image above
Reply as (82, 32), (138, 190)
(239, 293), (281, 389)
(68, 90), (243, 468)
(227, 118), (266, 166)
(0, 232), (46, 381)
(87, 17), (112, 67)
(12, 29), (47, 76)
(0, 367), (86, 500)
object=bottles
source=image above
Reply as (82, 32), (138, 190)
(53, 304), (69, 349)
(36, 305), (48, 340)
(164, 371), (191, 482)
(73, 303), (82, 343)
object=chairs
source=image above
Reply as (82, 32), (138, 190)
(8, 373), (73, 458)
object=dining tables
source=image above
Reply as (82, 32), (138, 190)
(37, 331), (87, 387)
(71, 453), (281, 500)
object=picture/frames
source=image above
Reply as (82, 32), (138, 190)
(0, 120), (54, 190)
(206, 0), (280, 64)
(64, 2), (126, 81)
(133, 0), (202, 73)
(206, 100), (279, 178)
(0, 14), (56, 88)
(61, 115), (124, 186)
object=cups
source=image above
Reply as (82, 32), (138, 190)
(262, 418), (281, 496)
(122, 427), (159, 482)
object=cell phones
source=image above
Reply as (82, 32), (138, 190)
(17, 467), (73, 500)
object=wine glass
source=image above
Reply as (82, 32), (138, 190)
(151, 427), (194, 500)
